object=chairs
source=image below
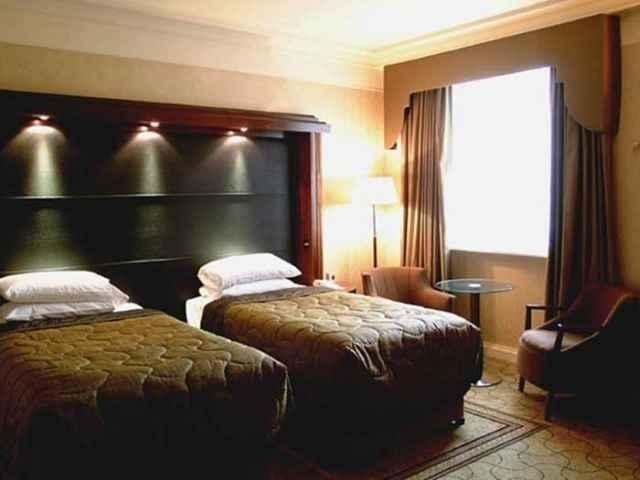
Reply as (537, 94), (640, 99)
(516, 281), (640, 420)
(360, 265), (455, 311)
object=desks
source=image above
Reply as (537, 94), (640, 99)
(434, 279), (513, 387)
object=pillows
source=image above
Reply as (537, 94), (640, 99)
(197, 252), (303, 299)
(0, 269), (128, 302)
